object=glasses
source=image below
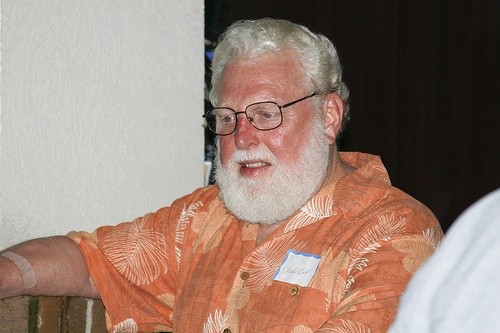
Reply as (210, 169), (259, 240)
(202, 92), (325, 136)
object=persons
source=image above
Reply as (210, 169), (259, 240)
(0, 17), (445, 333)
